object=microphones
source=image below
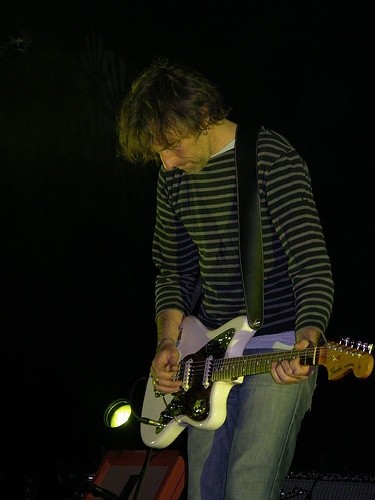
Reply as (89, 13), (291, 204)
(84, 482), (119, 500)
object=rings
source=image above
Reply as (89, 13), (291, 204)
(152, 380), (156, 385)
(155, 377), (159, 382)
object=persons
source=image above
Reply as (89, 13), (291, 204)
(118, 56), (334, 500)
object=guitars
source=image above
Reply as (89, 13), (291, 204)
(141, 314), (374, 449)
